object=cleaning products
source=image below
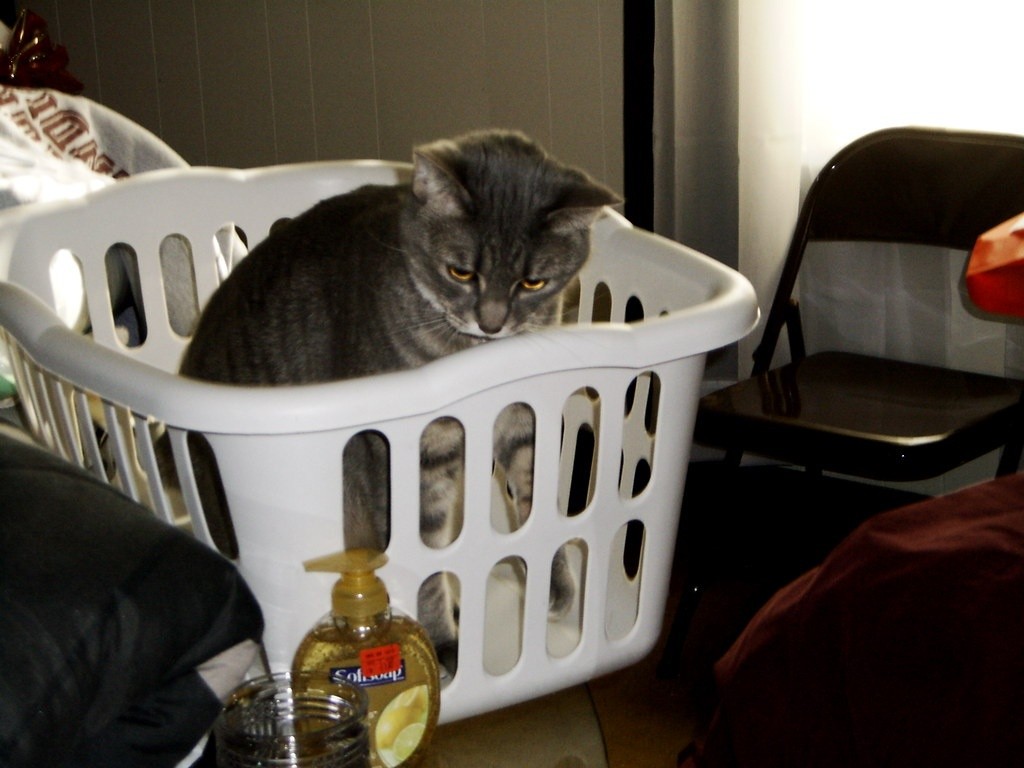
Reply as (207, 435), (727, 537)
(292, 549), (440, 768)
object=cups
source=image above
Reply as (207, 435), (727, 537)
(215, 670), (368, 768)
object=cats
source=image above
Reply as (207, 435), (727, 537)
(174, 126), (627, 689)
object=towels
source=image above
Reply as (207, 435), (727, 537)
(0, 86), (250, 336)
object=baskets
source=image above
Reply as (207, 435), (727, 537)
(0, 155), (761, 724)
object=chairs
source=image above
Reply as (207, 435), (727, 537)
(659, 128), (1022, 678)
